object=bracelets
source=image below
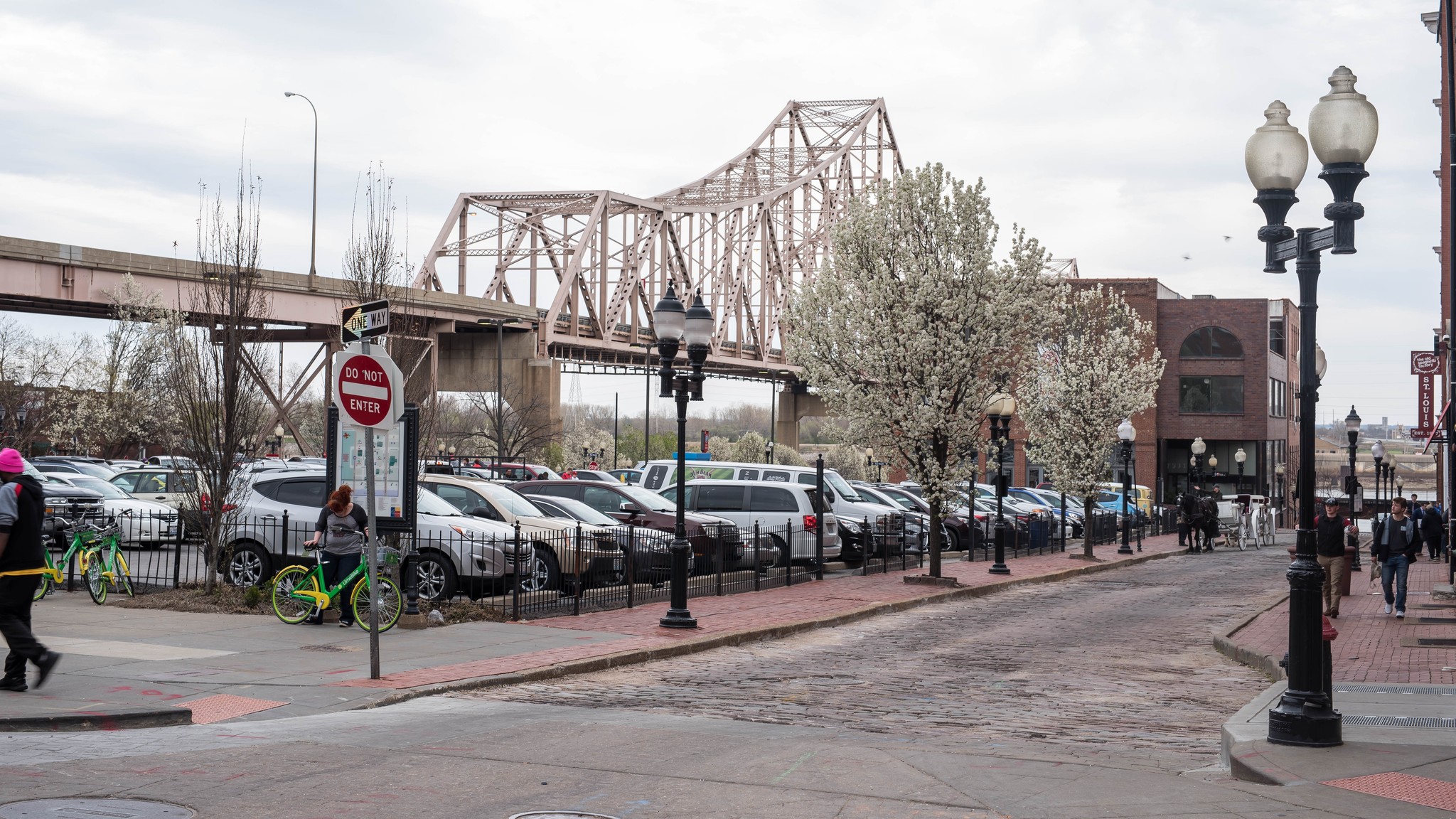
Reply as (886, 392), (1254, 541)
(365, 528), (368, 531)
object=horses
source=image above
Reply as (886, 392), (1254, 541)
(1174, 490), (1217, 556)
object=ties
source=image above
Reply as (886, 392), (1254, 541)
(1422, 510), (1425, 516)
(1412, 504), (1414, 513)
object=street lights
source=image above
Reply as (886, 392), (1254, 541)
(1369, 439), (1385, 550)
(651, 280), (714, 632)
(1344, 405), (1362, 571)
(265, 424), (285, 459)
(1209, 454), (1217, 487)
(1191, 437), (1206, 498)
(285, 90), (317, 276)
(1235, 448), (1247, 507)
(1290, 485), (1296, 530)
(1243, 63), (1378, 751)
(438, 441), (456, 466)
(1380, 448), (1404, 523)
(1275, 463), (1286, 529)
(1115, 417), (1136, 555)
(984, 385), (1016, 575)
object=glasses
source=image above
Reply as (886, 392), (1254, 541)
(1326, 500), (1335, 503)
(574, 472), (578, 474)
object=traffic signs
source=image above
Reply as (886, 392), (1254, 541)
(340, 299), (390, 342)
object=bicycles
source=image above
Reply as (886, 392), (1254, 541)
(30, 507), (98, 602)
(271, 527), (403, 634)
(83, 509), (136, 604)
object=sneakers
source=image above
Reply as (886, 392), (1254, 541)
(1426, 558), (1436, 562)
(1385, 603), (1393, 614)
(339, 621), (351, 627)
(301, 617), (316, 624)
(1436, 557), (1440, 561)
(1396, 610), (1404, 617)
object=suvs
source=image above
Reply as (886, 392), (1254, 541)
(105, 464), (215, 540)
(204, 469), (536, 605)
(21, 456), (106, 552)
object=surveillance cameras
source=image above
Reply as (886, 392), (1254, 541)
(1442, 335), (1451, 342)
(768, 442), (774, 448)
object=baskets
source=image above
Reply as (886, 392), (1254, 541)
(362, 545), (400, 572)
(93, 525), (127, 550)
(63, 524), (98, 545)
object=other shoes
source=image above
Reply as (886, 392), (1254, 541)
(1440, 550), (1448, 555)
(1331, 608), (1339, 618)
(0, 677), (28, 691)
(34, 651), (58, 689)
(1323, 609), (1331, 615)
(1416, 554), (1422, 556)
(1179, 543), (1188, 546)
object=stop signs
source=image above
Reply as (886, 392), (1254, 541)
(334, 351), (397, 430)
(588, 461), (599, 471)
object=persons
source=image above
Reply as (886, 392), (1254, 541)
(0, 448), (60, 693)
(1178, 493), (1188, 546)
(450, 454), (579, 482)
(1194, 484), (1223, 525)
(301, 484), (370, 627)
(1295, 494), (1449, 619)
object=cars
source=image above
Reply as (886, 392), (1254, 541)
(37, 472), (185, 549)
(29, 449), (1155, 595)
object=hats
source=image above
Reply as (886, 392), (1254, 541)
(1370, 562), (1381, 582)
(572, 471), (578, 475)
(1324, 498), (1339, 506)
(0, 448), (24, 473)
(1211, 484), (1219, 488)
(1426, 505), (1433, 509)
